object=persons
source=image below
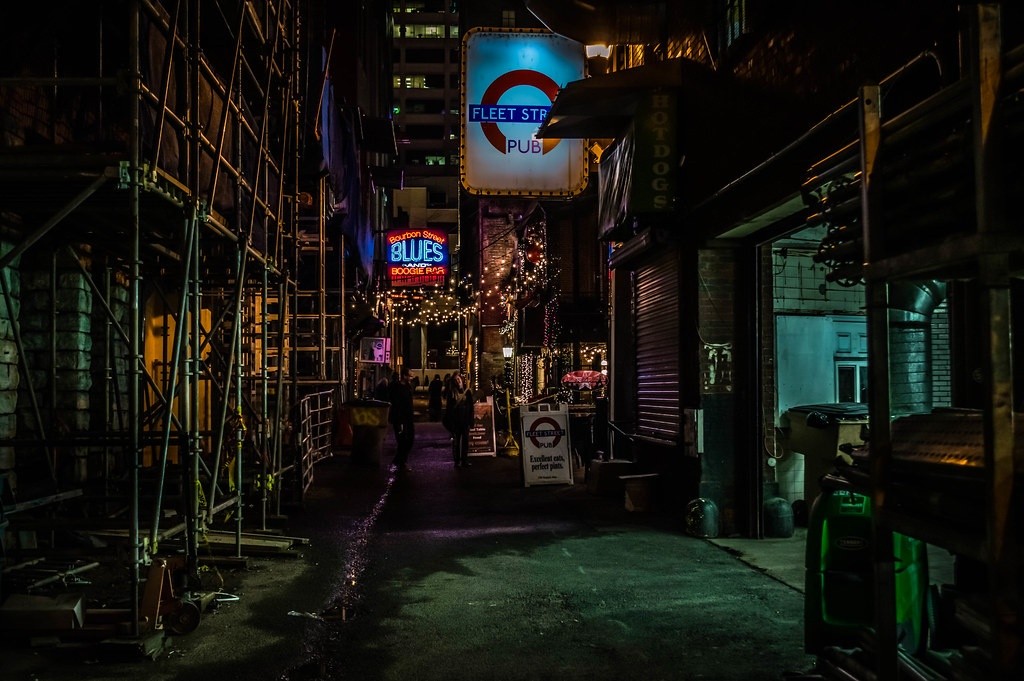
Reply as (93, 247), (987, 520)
(372, 340), (383, 361)
(389, 368), (414, 471)
(445, 371), (459, 400)
(425, 375), (429, 385)
(428, 374), (442, 410)
(445, 372), (474, 469)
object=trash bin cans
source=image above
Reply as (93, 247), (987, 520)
(785, 403), (871, 523)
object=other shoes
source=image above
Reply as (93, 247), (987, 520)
(454, 461), (461, 467)
(461, 460), (472, 467)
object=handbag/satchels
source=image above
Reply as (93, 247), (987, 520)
(442, 415), (456, 433)
